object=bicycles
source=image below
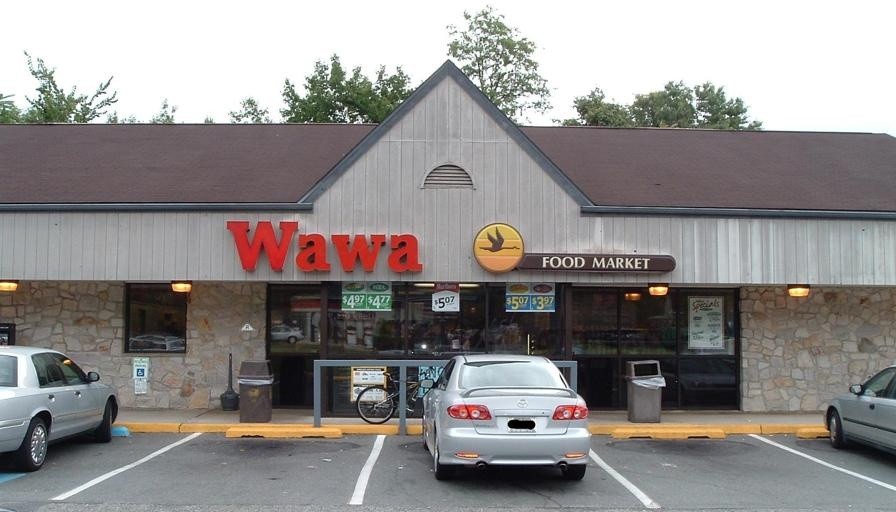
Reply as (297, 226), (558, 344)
(356, 367), (434, 424)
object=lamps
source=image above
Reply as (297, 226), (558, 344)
(170, 280), (192, 292)
(0, 280), (18, 292)
(624, 283), (669, 301)
(788, 284), (811, 297)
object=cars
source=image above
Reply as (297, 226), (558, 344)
(422, 353), (593, 480)
(824, 365), (896, 457)
(270, 326), (304, 343)
(0, 345), (119, 471)
(662, 358), (735, 404)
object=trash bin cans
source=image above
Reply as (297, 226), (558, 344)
(625, 360), (664, 424)
(238, 360), (275, 423)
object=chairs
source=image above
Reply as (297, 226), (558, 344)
(45, 362), (63, 382)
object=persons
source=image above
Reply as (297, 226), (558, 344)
(333, 325), (341, 343)
(372, 319), (416, 350)
